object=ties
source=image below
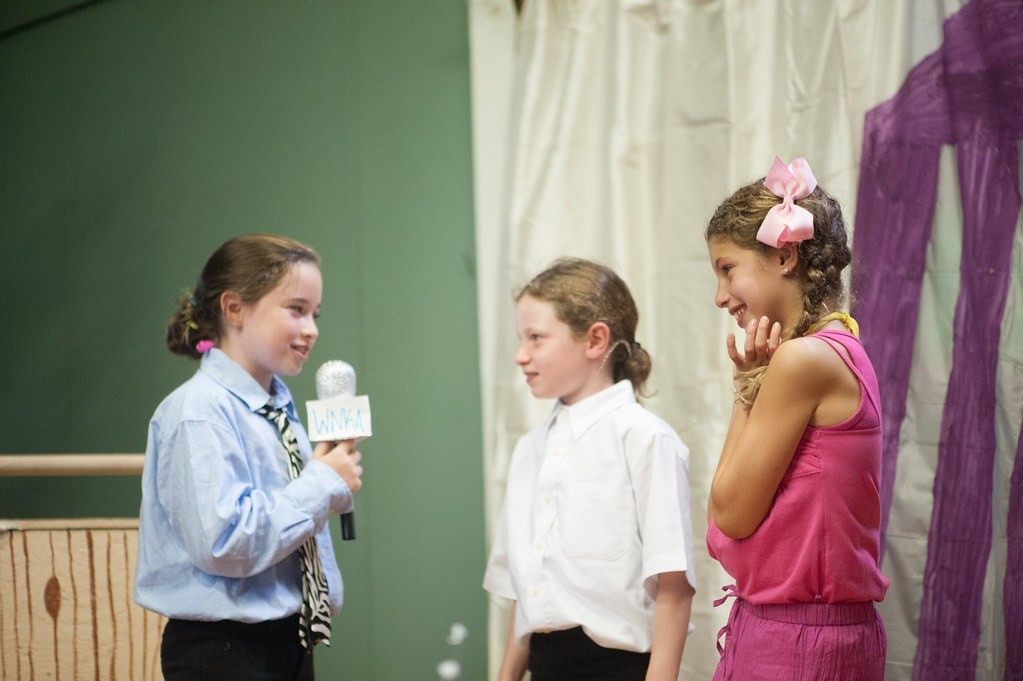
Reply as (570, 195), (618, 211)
(255, 404), (332, 654)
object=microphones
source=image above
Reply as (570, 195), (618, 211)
(305, 359), (373, 540)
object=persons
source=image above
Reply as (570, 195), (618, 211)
(479, 257), (697, 681)
(705, 156), (891, 681)
(133, 234), (363, 681)
